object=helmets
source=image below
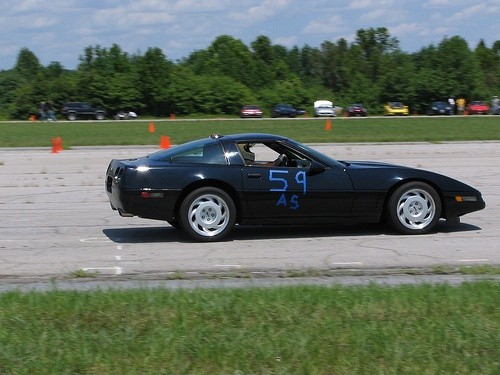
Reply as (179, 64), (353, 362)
(239, 143), (255, 161)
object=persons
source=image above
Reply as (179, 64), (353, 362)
(237, 143), (288, 166)
(491, 96), (500, 115)
(449, 96), (466, 115)
(40, 101), (56, 121)
(119, 111), (137, 120)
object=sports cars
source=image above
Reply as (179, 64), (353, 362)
(107, 133), (486, 243)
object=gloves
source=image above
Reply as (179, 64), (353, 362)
(279, 154), (286, 161)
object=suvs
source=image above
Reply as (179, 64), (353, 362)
(62, 102), (108, 121)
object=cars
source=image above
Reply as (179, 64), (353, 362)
(241, 105), (263, 118)
(271, 104), (306, 118)
(384, 102), (410, 116)
(465, 100), (490, 116)
(426, 101), (453, 116)
(313, 100), (336, 118)
(343, 103), (368, 117)
